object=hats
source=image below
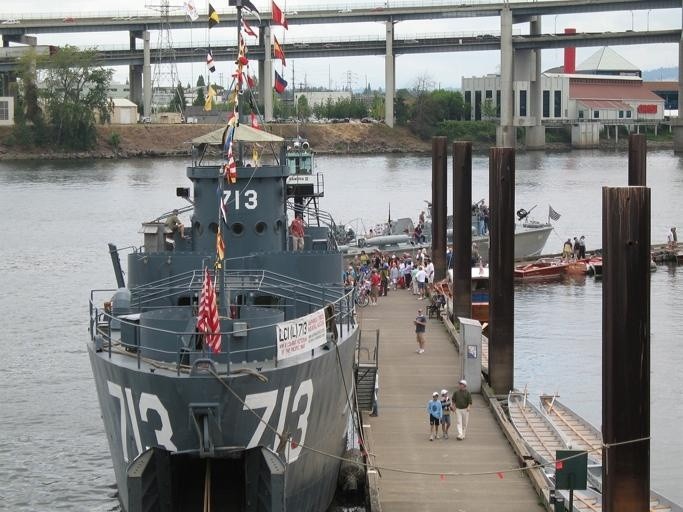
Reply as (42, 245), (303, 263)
(297, 213), (302, 218)
(432, 392), (439, 397)
(441, 389), (448, 395)
(459, 380), (467, 387)
(349, 247), (429, 273)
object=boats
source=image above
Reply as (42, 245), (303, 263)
(585, 455), (683, 512)
(539, 465), (603, 511)
(336, 196), (561, 281)
(506, 388), (575, 470)
(443, 264), (489, 324)
(536, 386), (605, 464)
(514, 240), (683, 285)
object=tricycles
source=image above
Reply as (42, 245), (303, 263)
(355, 292), (370, 307)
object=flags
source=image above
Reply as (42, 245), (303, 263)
(213, 0), (262, 269)
(186, 1), (199, 22)
(548, 206), (562, 222)
(205, 43), (216, 74)
(272, 70), (288, 95)
(207, 2), (220, 29)
(272, 35), (286, 68)
(271, 0), (289, 32)
(201, 83), (217, 113)
(196, 268), (221, 355)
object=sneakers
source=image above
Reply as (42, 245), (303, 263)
(456, 432), (465, 440)
(416, 348), (424, 354)
(369, 302), (378, 306)
(429, 431), (449, 441)
(408, 287), (426, 300)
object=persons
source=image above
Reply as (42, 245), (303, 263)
(341, 200), (490, 315)
(576, 235), (586, 260)
(412, 308), (427, 354)
(288, 211), (308, 253)
(451, 379), (472, 441)
(165, 208), (186, 239)
(438, 388), (456, 440)
(571, 237), (579, 259)
(426, 391), (442, 442)
(562, 237), (572, 259)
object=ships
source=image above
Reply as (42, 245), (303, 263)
(83, 0), (381, 512)
